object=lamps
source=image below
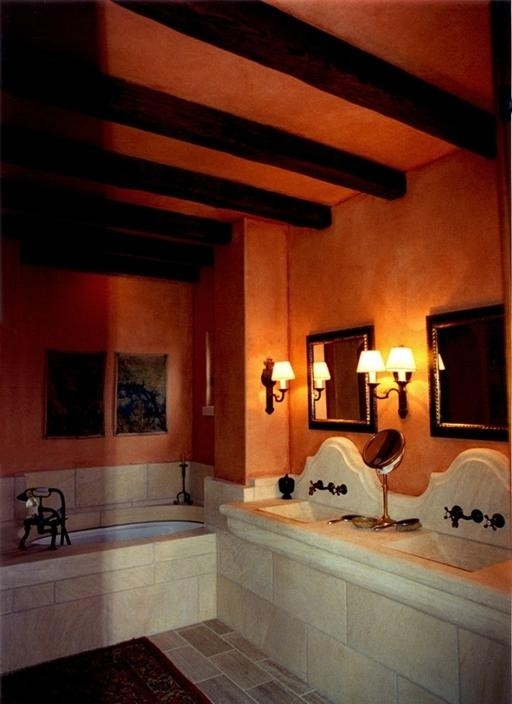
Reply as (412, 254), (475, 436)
(314, 362), (331, 401)
(356, 346), (415, 419)
(261, 359), (295, 414)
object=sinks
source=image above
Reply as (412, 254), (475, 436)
(402, 531), (511, 570)
(263, 502), (348, 524)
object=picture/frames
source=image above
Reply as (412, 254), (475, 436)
(113, 352), (169, 436)
(43, 349), (107, 440)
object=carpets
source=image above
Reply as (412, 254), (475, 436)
(1, 636), (215, 704)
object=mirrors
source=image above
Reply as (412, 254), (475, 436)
(306, 323), (378, 434)
(426, 303), (508, 442)
(362, 427), (406, 528)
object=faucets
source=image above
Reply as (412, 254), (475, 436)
(444, 507), (478, 528)
(309, 480), (333, 495)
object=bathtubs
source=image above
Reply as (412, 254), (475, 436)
(19, 520), (207, 545)
